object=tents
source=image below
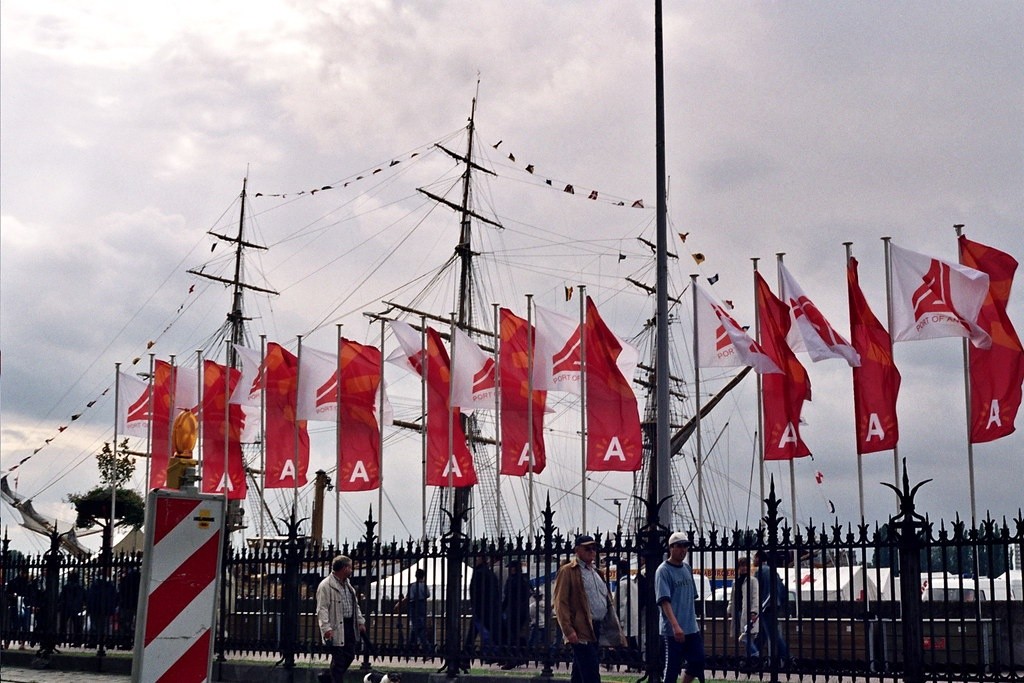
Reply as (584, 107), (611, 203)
(614, 556), (1024, 639)
(371, 556), (478, 598)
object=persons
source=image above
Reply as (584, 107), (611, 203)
(530, 596), (554, 645)
(488, 551), (511, 625)
(752, 549), (802, 672)
(316, 554), (366, 683)
(0, 566), (136, 651)
(726, 557), (759, 668)
(460, 553), (503, 670)
(553, 536), (647, 683)
(503, 558), (532, 669)
(392, 568), (432, 662)
(655, 531), (706, 683)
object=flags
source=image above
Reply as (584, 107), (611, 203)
(777, 261), (862, 367)
(957, 234), (1024, 444)
(754, 270), (814, 461)
(116, 291), (642, 496)
(847, 257), (902, 454)
(888, 242), (992, 351)
(666, 210), (785, 374)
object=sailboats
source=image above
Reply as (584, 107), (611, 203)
(1, 99), (1023, 669)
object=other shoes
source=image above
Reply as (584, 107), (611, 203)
(318, 672), (326, 683)
(501, 663), (515, 670)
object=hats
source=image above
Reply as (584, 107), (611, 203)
(575, 536), (599, 546)
(506, 560), (522, 568)
(669, 532), (691, 546)
